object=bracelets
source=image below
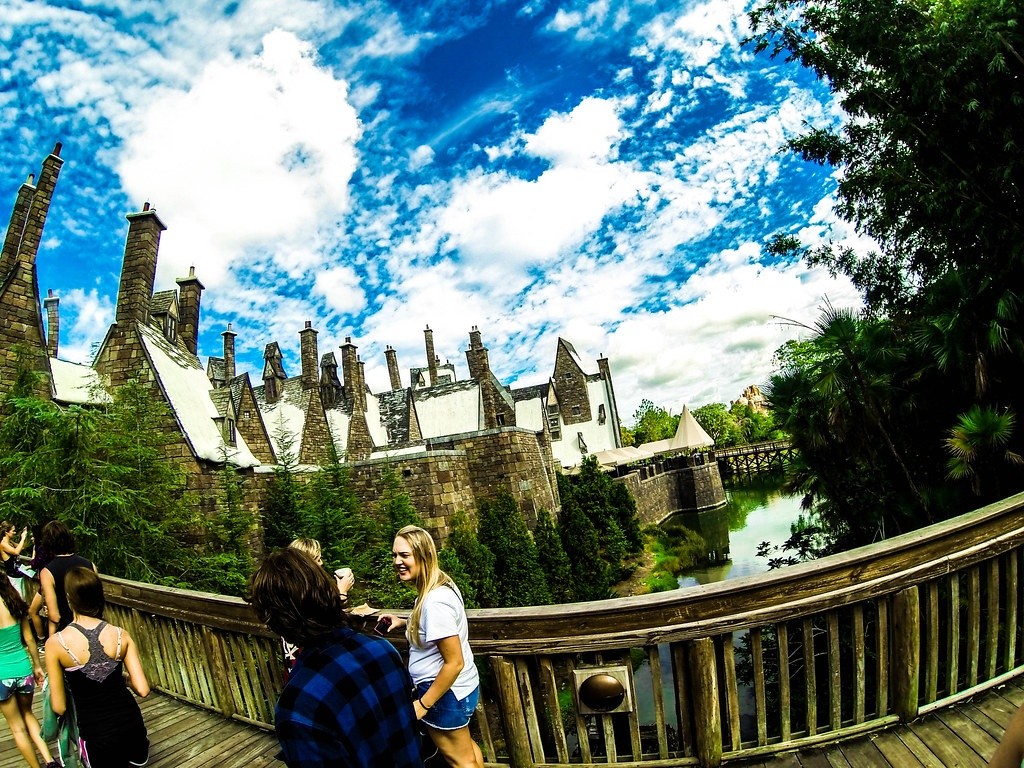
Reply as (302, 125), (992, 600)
(418, 699), (430, 710)
(336, 593), (348, 597)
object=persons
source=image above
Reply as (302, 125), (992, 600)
(45, 567), (151, 768)
(0, 519), (98, 655)
(0, 572), (61, 768)
(377, 525), (485, 768)
(287, 537), (356, 614)
(246, 547), (451, 768)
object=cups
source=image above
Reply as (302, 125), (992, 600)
(334, 567), (354, 592)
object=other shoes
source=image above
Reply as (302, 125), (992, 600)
(36, 640), (44, 646)
(47, 756), (64, 768)
